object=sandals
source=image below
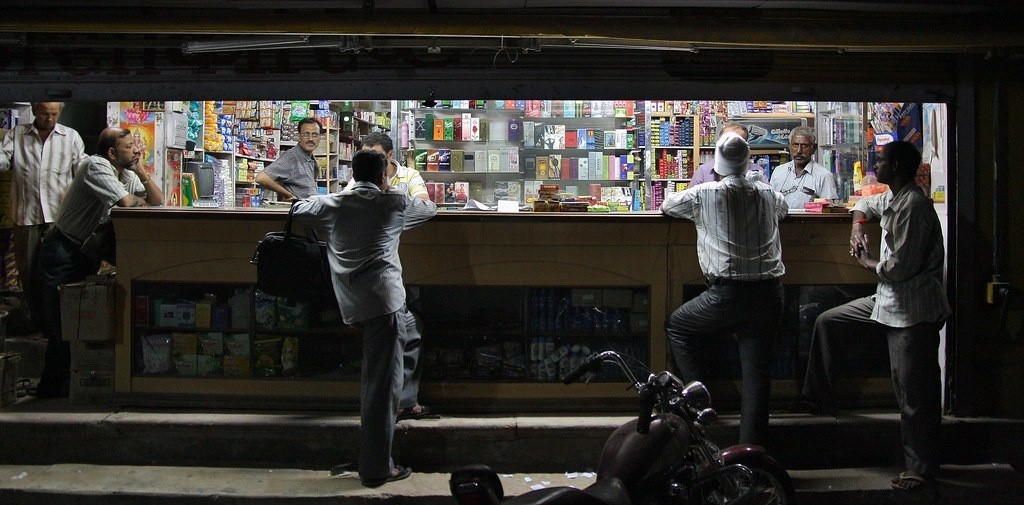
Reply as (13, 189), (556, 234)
(398, 405), (433, 419)
(361, 465), (412, 487)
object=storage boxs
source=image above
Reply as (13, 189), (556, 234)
(523, 150), (641, 180)
(822, 206), (848, 213)
(813, 198), (831, 202)
(135, 295), (253, 379)
(494, 180), (521, 204)
(406, 146), (519, 172)
(651, 181), (688, 210)
(524, 181), (647, 212)
(402, 100), (486, 110)
(571, 287), (650, 312)
(409, 113), (488, 141)
(489, 97), (635, 118)
(0, 352), (21, 408)
(727, 101), (811, 118)
(68, 341), (114, 408)
(489, 118), (519, 141)
(233, 101), (391, 208)
(804, 202), (828, 213)
(425, 181), (483, 204)
(651, 117), (694, 147)
(748, 155), (790, 181)
(872, 101), (923, 150)
(57, 276), (115, 341)
(651, 101), (697, 115)
(5, 338), (49, 378)
(523, 120), (637, 149)
(651, 149), (694, 180)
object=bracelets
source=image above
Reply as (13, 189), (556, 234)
(852, 219), (866, 224)
(141, 172), (151, 184)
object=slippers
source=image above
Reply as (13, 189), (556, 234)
(892, 470), (933, 493)
(789, 399), (841, 416)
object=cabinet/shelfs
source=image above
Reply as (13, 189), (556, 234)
(668, 213), (897, 406)
(108, 206), (363, 403)
(520, 115), (645, 209)
(694, 100), (819, 184)
(399, 210), (668, 415)
(180, 101), (281, 208)
(281, 116), (339, 194)
(819, 102), (868, 204)
(648, 100), (701, 211)
(340, 111), (392, 194)
(396, 100), (524, 210)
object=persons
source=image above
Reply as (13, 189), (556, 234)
(291, 148), (437, 487)
(787, 139), (952, 492)
(30, 128), (165, 401)
(688, 124), (772, 189)
(0, 101), (91, 341)
(254, 116), (324, 203)
(770, 126), (840, 209)
(342, 131), (430, 200)
(660, 132), (788, 451)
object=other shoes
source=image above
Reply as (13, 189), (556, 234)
(36, 381), (71, 399)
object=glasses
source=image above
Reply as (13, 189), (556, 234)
(299, 133), (321, 139)
(780, 175), (805, 196)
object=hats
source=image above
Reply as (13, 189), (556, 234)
(713, 131), (750, 176)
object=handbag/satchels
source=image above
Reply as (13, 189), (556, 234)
(251, 200), (338, 309)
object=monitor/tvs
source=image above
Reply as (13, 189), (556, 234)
(183, 162), (214, 200)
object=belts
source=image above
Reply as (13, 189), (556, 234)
(52, 226), (90, 254)
(714, 277), (781, 287)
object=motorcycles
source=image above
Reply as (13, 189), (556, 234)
(449, 350), (799, 505)
